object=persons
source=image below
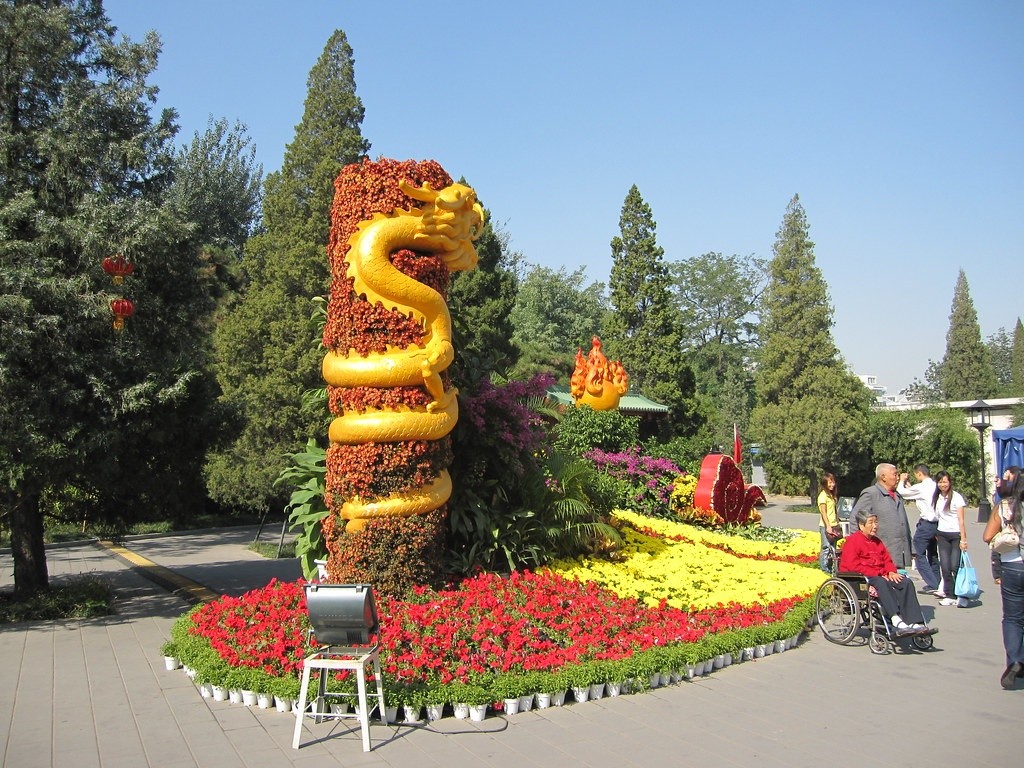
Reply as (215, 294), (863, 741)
(816, 473), (840, 575)
(933, 471), (969, 606)
(983, 465), (1024, 690)
(841, 506), (939, 638)
(897, 463), (942, 594)
(850, 462), (917, 572)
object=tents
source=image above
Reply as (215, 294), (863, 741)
(991, 426), (1024, 478)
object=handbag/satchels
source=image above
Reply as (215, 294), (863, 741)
(988, 498), (1019, 553)
(825, 525), (844, 545)
(954, 550), (979, 600)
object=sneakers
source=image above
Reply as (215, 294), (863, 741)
(939, 597), (956, 606)
(956, 598), (969, 608)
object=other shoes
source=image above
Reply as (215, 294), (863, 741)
(917, 589), (926, 593)
(928, 590), (937, 594)
(896, 621), (929, 638)
(910, 623), (938, 637)
(1000, 661), (1022, 690)
(994, 578), (1000, 583)
(933, 591), (947, 598)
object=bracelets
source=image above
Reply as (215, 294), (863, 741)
(960, 540), (968, 544)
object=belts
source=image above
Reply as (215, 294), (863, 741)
(921, 518), (936, 524)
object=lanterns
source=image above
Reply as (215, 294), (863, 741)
(109, 298), (134, 329)
(101, 254), (136, 283)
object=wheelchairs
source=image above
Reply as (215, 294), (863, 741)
(816, 546), (933, 654)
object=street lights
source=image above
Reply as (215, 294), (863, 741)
(971, 398), (992, 523)
